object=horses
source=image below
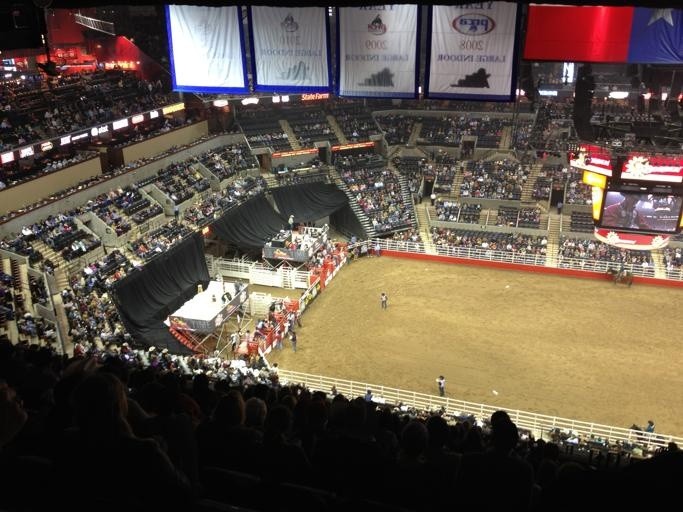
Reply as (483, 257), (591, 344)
(606, 268), (634, 287)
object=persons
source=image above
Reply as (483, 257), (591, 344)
(365, 60), (682, 287)
(379, 291), (388, 307)
(0, 63), (363, 366)
(1, 365), (682, 512)
